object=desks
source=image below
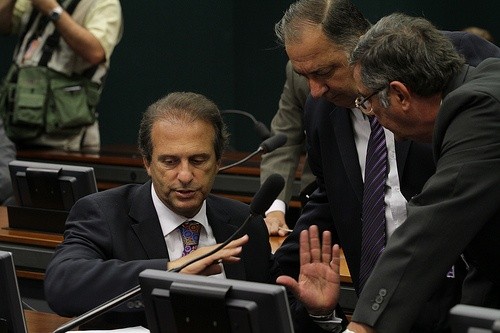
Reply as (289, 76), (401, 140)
(0, 145), (358, 333)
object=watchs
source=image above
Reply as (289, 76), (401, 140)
(50, 7), (61, 19)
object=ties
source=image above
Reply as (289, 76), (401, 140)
(359, 113), (387, 291)
(179, 221), (202, 257)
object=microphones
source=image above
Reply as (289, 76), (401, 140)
(220, 134), (288, 171)
(222, 110), (272, 139)
(168, 173), (286, 273)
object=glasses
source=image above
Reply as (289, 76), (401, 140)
(355, 79), (394, 115)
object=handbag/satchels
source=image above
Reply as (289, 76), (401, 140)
(1, 67), (102, 150)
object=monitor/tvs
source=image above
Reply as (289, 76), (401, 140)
(9, 160), (98, 211)
(0, 250), (28, 333)
(139, 268), (293, 333)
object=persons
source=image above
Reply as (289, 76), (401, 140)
(44, 92), (348, 333)
(0, 0), (123, 157)
(275, 0), (500, 333)
(346, 13), (500, 333)
